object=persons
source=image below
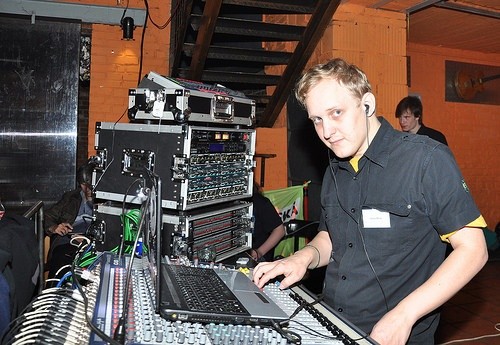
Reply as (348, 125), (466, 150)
(44, 162), (94, 287)
(394, 96), (448, 146)
(252, 57), (489, 345)
(253, 180), (285, 261)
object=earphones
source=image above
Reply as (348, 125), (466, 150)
(365, 105), (369, 113)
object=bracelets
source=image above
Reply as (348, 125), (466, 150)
(303, 245), (320, 268)
(254, 250), (258, 259)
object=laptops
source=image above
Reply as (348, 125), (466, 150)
(142, 166), (290, 327)
